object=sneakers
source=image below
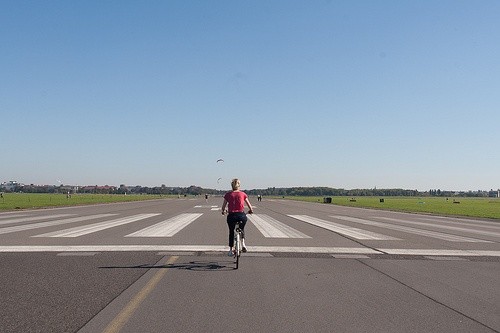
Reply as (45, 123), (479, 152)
(229, 251), (234, 256)
(242, 246), (247, 253)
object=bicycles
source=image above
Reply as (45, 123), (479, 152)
(222, 210), (253, 269)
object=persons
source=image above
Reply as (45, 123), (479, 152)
(222, 178), (254, 257)
